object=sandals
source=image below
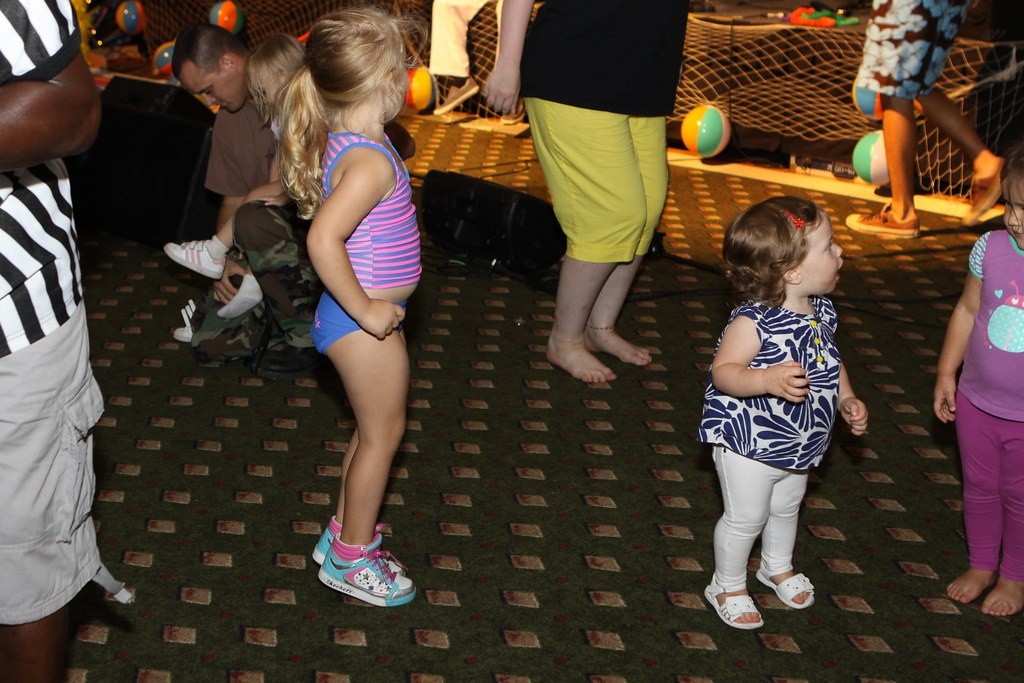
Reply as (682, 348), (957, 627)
(704, 574), (764, 629)
(756, 564), (815, 609)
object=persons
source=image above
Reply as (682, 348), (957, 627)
(694, 197), (867, 631)
(428, 0), (692, 383)
(271, 4), (421, 607)
(0, 0), (133, 683)
(935, 141), (1024, 616)
(845, 0), (1004, 239)
(164, 22), (416, 381)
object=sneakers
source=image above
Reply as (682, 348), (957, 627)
(963, 158), (1006, 225)
(845, 202), (921, 239)
(312, 515), (408, 576)
(164, 240), (226, 279)
(318, 524), (416, 606)
(172, 299), (197, 342)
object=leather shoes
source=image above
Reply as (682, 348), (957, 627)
(255, 342), (326, 381)
(189, 346), (241, 363)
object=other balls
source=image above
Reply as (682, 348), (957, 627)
(153, 42), (173, 76)
(404, 66), (437, 112)
(116, 1), (147, 35)
(851, 129), (891, 186)
(680, 105), (732, 157)
(209, 1), (244, 33)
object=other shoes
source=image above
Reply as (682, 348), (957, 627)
(501, 103), (526, 124)
(433, 76), (480, 113)
